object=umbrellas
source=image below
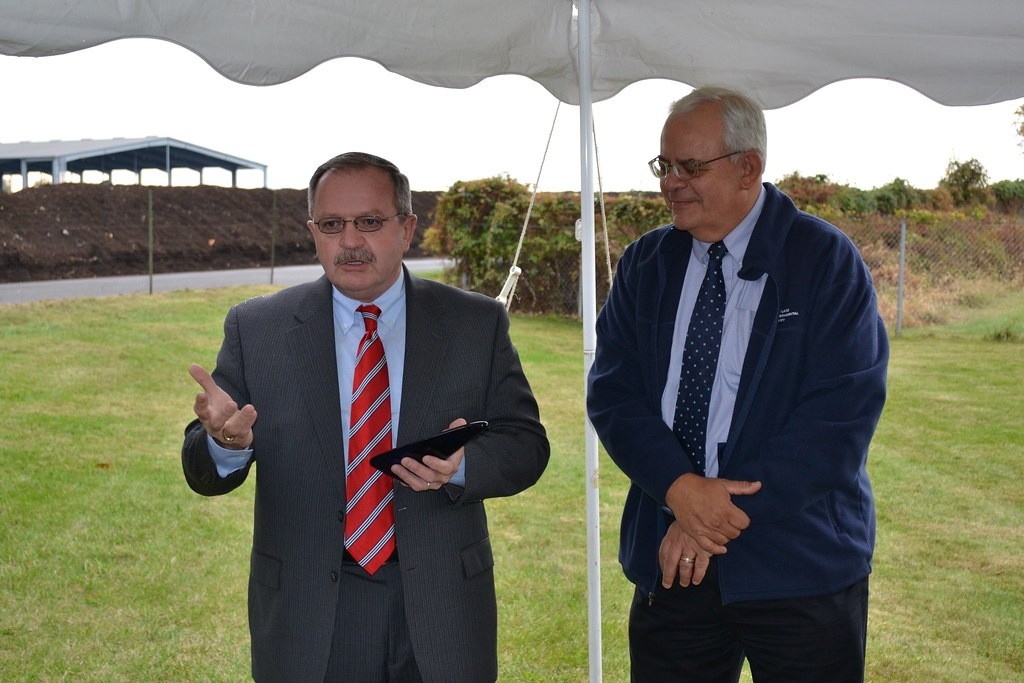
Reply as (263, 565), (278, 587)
(0, 0), (1023, 682)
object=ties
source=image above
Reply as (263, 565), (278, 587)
(343, 304), (396, 575)
(672, 240), (729, 479)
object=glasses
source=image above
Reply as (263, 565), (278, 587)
(312, 212), (406, 235)
(648, 151), (742, 179)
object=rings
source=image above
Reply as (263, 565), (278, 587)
(223, 431), (236, 442)
(427, 483), (431, 490)
(680, 558), (694, 564)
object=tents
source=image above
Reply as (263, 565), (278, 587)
(0, 135), (269, 188)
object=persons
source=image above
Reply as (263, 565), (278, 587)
(585, 82), (890, 683)
(179, 149), (551, 683)
